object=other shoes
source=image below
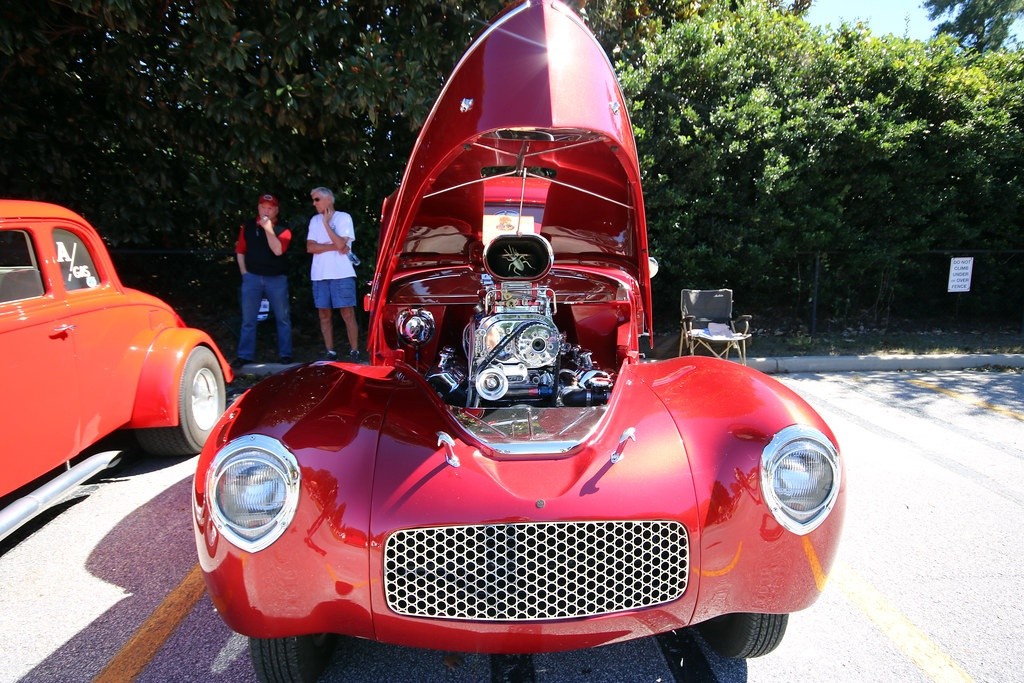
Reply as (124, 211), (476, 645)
(321, 353), (338, 361)
(277, 357), (292, 364)
(231, 358), (252, 367)
(348, 350), (360, 360)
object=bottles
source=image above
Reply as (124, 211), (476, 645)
(346, 251), (361, 266)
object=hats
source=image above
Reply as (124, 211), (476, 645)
(258, 195), (278, 206)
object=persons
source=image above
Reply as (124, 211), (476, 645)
(307, 186), (360, 362)
(230, 194), (293, 369)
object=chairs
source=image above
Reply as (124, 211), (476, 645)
(678, 289), (752, 366)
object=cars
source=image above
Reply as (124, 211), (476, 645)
(192, 1), (846, 683)
(0, 200), (235, 558)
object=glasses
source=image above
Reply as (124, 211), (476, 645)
(312, 198), (327, 202)
